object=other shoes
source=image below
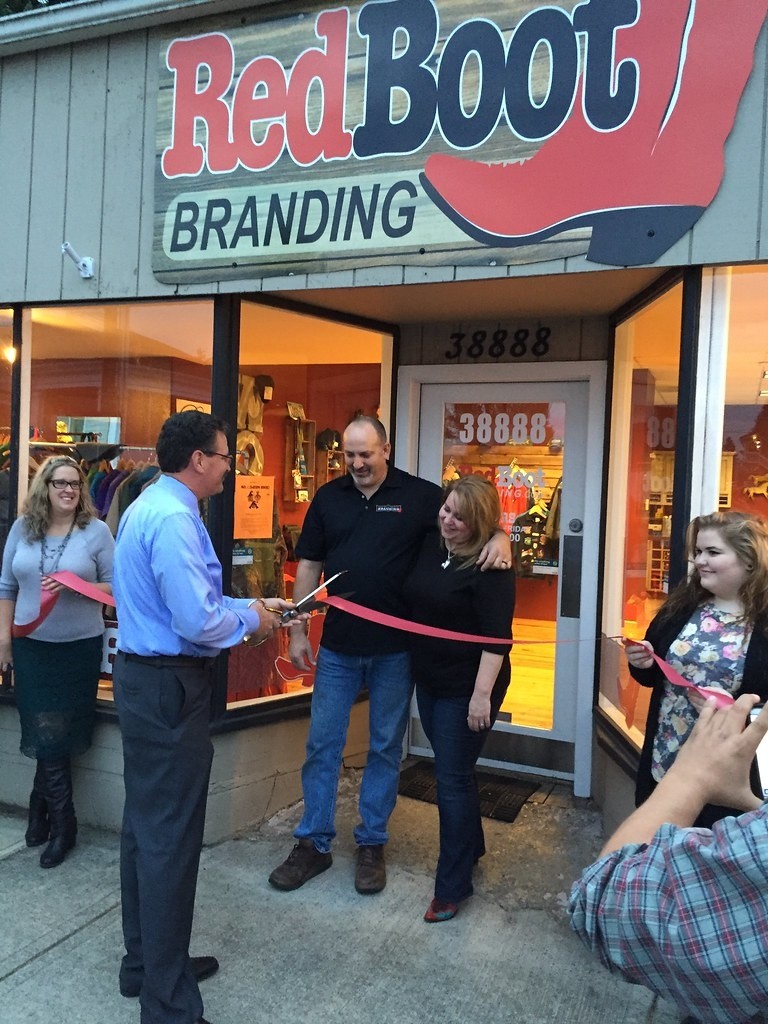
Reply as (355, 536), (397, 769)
(191, 957), (220, 982)
(266, 838), (334, 890)
(356, 842), (389, 895)
(425, 899), (460, 922)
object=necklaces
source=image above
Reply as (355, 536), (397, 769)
(39, 521), (75, 576)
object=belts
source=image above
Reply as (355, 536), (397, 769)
(126, 653), (213, 667)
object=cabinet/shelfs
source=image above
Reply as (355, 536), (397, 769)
(644, 451), (733, 594)
(283, 418), (345, 503)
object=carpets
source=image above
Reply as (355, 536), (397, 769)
(397, 760), (541, 822)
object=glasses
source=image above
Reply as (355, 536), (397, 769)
(44, 477), (85, 492)
(200, 450), (233, 467)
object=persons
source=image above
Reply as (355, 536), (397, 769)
(108, 410), (312, 1024)
(269, 416), (512, 893)
(620, 512), (768, 829)
(411, 474), (517, 923)
(565, 693), (768, 1024)
(0, 455), (116, 867)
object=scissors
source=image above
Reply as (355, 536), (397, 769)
(243, 570), (356, 648)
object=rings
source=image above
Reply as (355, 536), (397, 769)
(502, 561), (507, 565)
(480, 723), (484, 727)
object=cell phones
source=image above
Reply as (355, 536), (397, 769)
(747, 703), (768, 801)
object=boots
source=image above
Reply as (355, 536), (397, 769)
(25, 766), (54, 847)
(38, 764), (78, 870)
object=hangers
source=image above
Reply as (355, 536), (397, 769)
(495, 458), (534, 483)
(529, 493), (549, 518)
(443, 458), (471, 479)
(1, 429), (175, 473)
